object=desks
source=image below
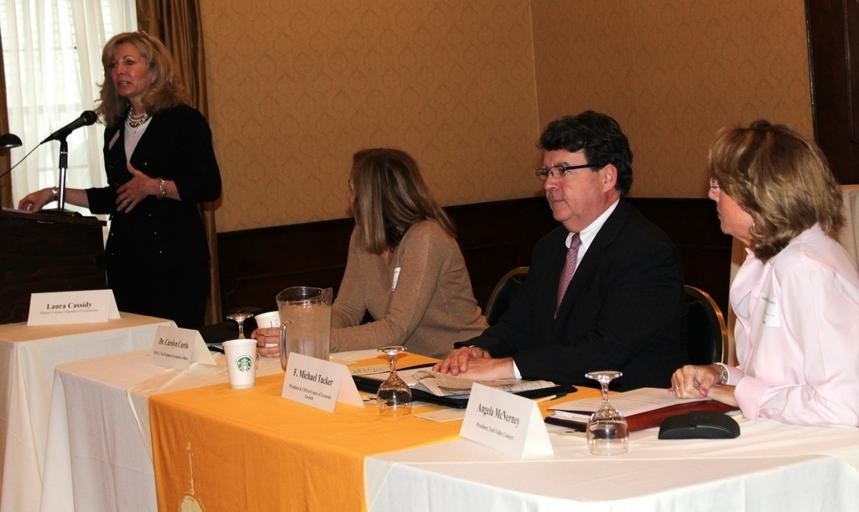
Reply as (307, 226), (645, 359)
(0, 312), (179, 512)
(39, 350), (857, 512)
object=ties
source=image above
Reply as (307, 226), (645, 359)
(554, 233), (583, 318)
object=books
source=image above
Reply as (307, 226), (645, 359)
(353, 363), (577, 409)
(543, 388), (740, 437)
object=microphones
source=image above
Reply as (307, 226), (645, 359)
(41, 110), (97, 144)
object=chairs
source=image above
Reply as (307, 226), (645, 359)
(484, 266), (528, 321)
(683, 285), (726, 362)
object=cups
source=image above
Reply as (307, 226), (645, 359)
(223, 338), (257, 388)
(254, 310), (280, 358)
(277, 286), (333, 370)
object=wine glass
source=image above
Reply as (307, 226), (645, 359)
(227, 312), (254, 339)
(585, 371), (629, 455)
(377, 346), (412, 416)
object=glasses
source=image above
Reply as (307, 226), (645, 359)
(533, 162), (605, 180)
(709, 176), (723, 194)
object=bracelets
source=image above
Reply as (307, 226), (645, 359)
(50, 186), (60, 201)
(158, 179), (168, 201)
(720, 365), (728, 385)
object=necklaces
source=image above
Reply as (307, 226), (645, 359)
(127, 107), (150, 128)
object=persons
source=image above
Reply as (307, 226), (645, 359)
(249, 148), (490, 359)
(433, 111), (715, 390)
(672, 118), (858, 428)
(18, 31), (222, 330)
